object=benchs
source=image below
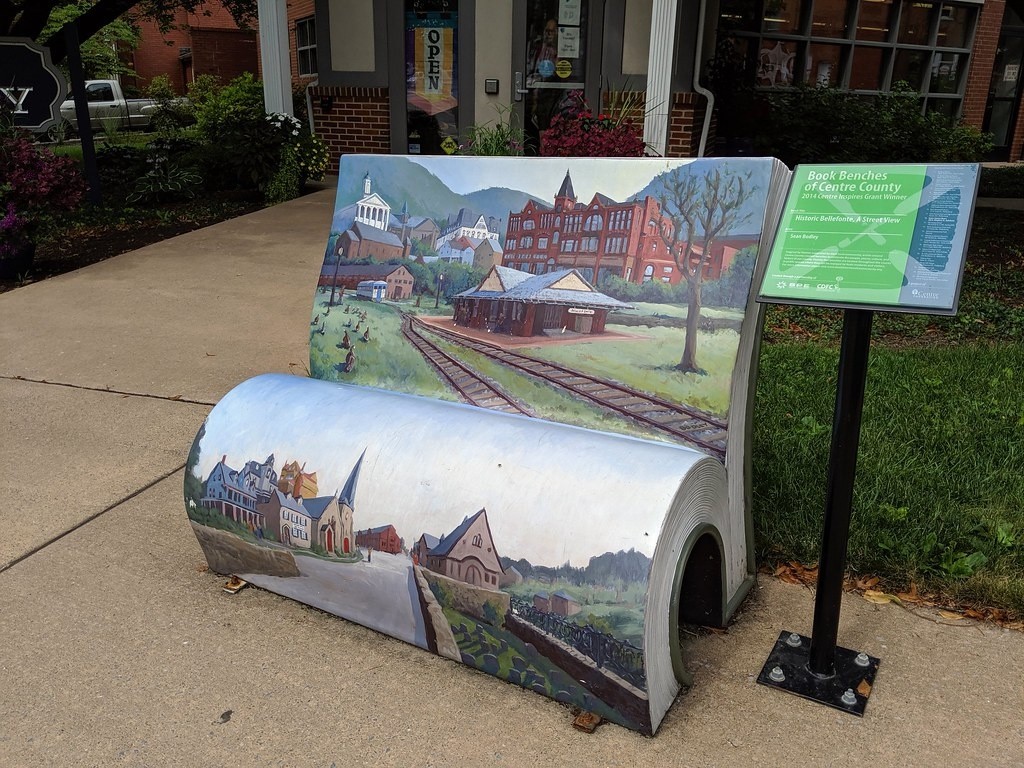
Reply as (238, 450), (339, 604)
(181, 155), (793, 737)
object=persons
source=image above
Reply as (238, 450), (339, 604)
(532, 17), (564, 131)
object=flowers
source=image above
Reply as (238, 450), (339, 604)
(541, 76), (667, 157)
(456, 101), (537, 157)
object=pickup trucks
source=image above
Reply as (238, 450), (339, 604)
(36, 79), (193, 143)
